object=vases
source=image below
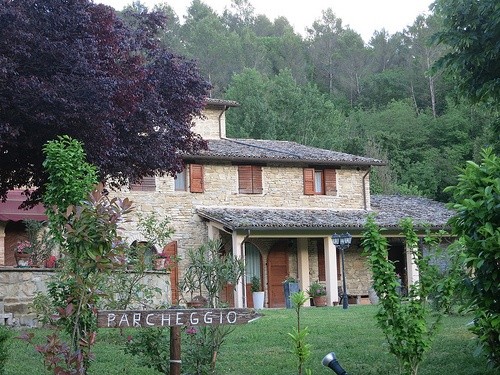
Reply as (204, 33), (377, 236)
(14, 252), (30, 266)
(310, 294), (327, 307)
(151, 259), (167, 271)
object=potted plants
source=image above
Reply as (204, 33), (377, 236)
(250, 276), (266, 309)
(281, 276), (299, 309)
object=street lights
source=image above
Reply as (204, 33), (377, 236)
(331, 232), (352, 309)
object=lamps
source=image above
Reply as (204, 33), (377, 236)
(322, 352), (346, 375)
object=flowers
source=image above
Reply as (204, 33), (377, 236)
(149, 253), (170, 265)
(12, 239), (33, 255)
(307, 281), (327, 297)
